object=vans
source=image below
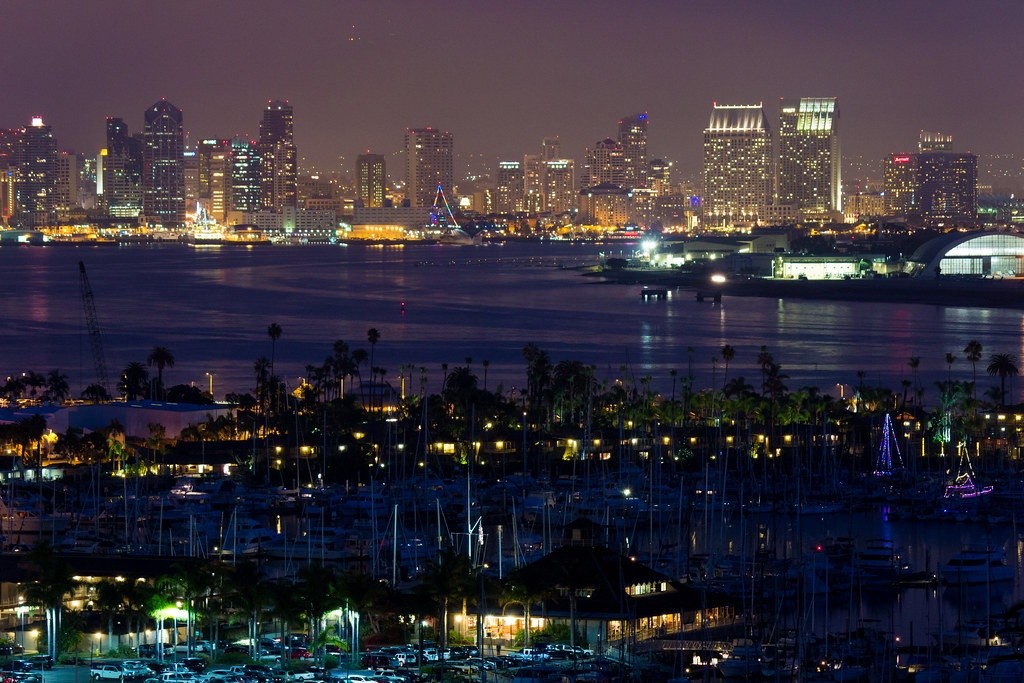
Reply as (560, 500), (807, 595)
(28, 654), (54, 670)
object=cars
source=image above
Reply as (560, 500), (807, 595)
(2, 660), (33, 673)
(0, 670), (45, 683)
(136, 631), (598, 683)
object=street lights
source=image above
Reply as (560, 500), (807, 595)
(17, 595), (25, 661)
(31, 630), (40, 654)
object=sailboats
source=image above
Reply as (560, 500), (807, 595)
(0, 399), (1022, 572)
(687, 506), (1024, 683)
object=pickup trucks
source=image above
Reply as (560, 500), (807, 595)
(90, 662), (136, 682)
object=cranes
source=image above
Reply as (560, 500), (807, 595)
(70, 257), (117, 408)
